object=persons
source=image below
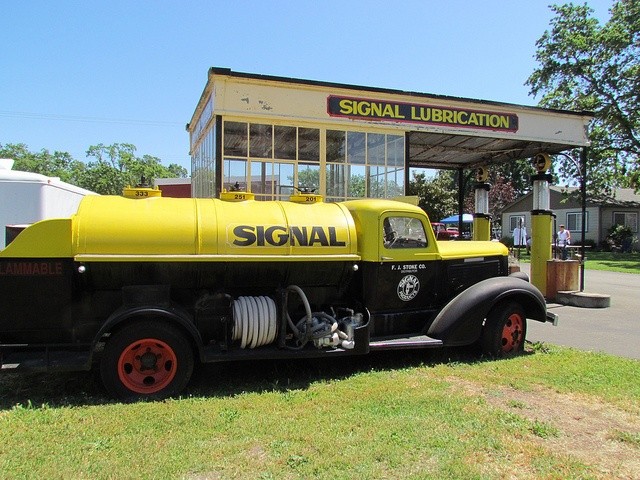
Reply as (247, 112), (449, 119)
(558, 224), (571, 260)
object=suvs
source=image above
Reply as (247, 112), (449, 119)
(431, 222), (460, 240)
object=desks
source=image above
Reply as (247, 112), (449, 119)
(552, 244), (591, 260)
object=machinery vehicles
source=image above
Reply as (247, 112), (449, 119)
(1, 185), (559, 402)
